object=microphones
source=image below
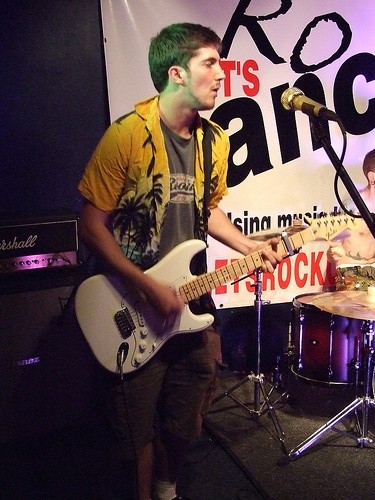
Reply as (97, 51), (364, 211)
(281, 87), (340, 121)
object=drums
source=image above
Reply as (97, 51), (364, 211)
(290, 292), (375, 385)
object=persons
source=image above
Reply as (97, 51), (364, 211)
(75, 23), (282, 500)
(339, 148), (375, 232)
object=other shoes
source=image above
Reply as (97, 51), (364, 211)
(150, 484), (186, 500)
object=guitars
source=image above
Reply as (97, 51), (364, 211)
(74, 210), (356, 375)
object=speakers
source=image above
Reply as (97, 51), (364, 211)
(0, 272), (110, 444)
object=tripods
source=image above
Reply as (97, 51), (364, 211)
(287, 320), (375, 462)
(205, 227), (293, 455)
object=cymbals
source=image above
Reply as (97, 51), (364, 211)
(313, 291), (375, 320)
